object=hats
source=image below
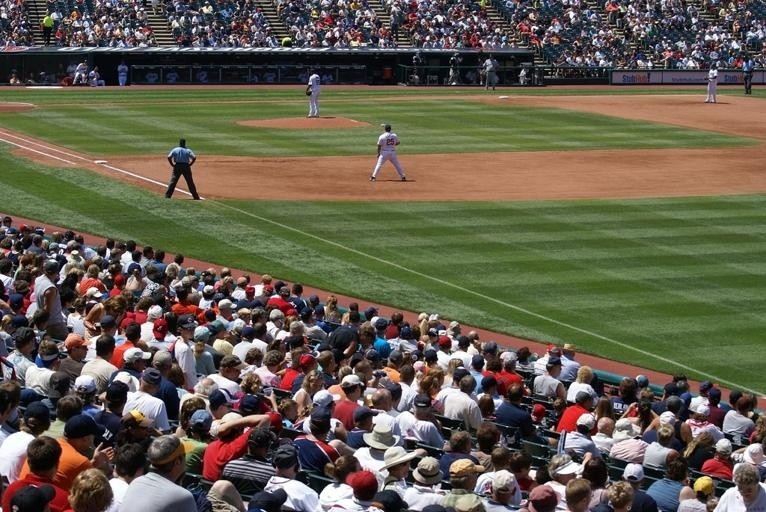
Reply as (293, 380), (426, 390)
(384, 124), (391, 131)
(0, 225), (766, 512)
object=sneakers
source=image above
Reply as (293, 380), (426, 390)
(369, 176), (376, 182)
(402, 176), (406, 181)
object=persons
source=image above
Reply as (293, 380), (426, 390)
(1, 214), (766, 511)
(483, 50), (500, 92)
(742, 54), (756, 94)
(703, 61), (720, 104)
(368, 124), (406, 181)
(305, 70), (320, 118)
(0, 2), (766, 86)
(165, 137), (206, 200)
